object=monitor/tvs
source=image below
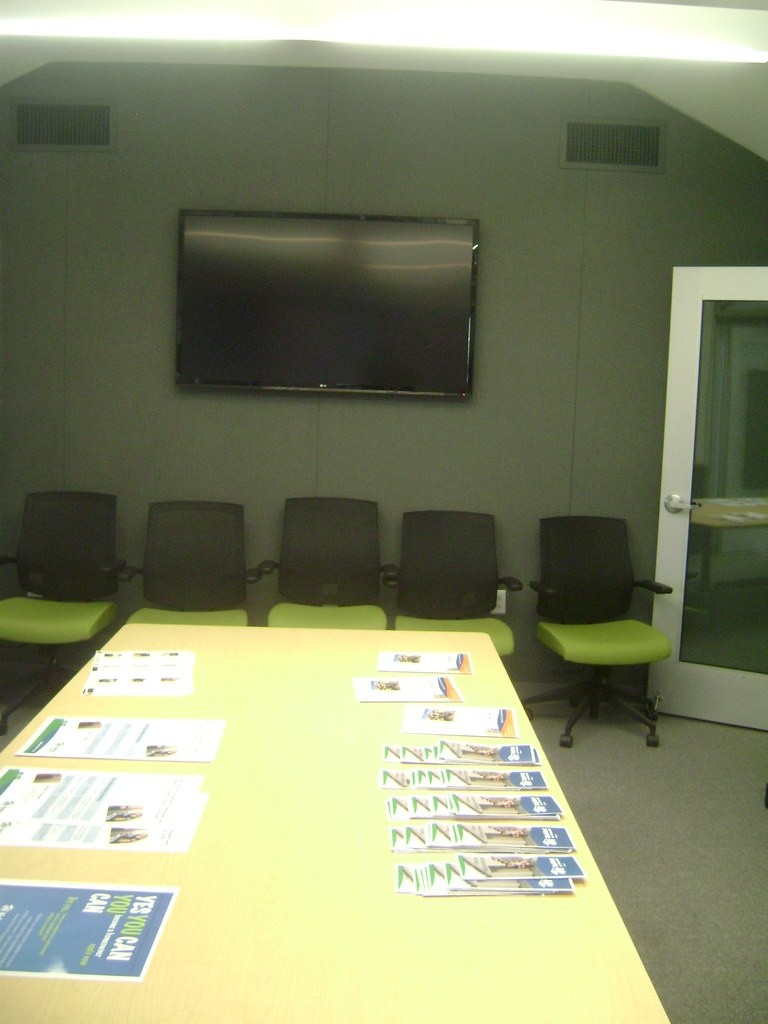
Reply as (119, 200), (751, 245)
(177, 208), (479, 400)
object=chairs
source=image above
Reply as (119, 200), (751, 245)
(522, 516), (673, 747)
(258, 496), (398, 630)
(117, 501), (261, 627)
(0, 492), (125, 736)
(381, 510), (524, 658)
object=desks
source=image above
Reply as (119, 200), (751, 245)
(0, 622), (671, 1024)
